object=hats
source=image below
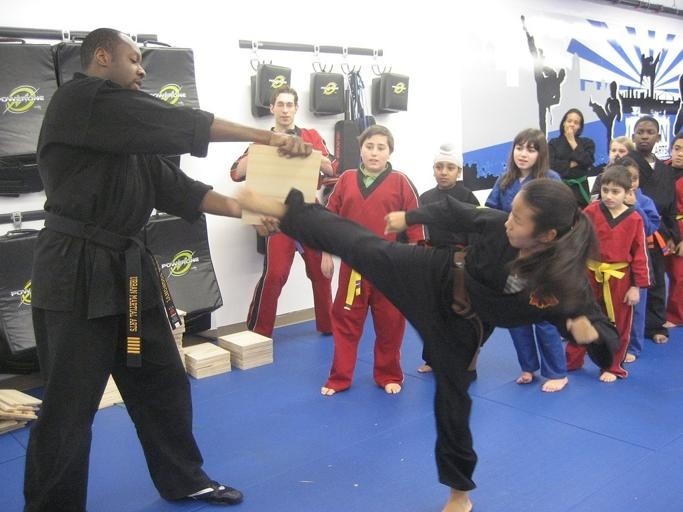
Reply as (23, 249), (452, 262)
(432, 143), (462, 169)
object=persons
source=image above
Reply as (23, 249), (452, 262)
(485, 128), (568, 393)
(591, 116), (683, 362)
(238, 178), (620, 511)
(566, 165), (650, 383)
(21, 28), (311, 512)
(230, 87), (333, 337)
(320, 125), (430, 395)
(548, 109), (596, 211)
(416, 145), (480, 373)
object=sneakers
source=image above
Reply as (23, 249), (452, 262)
(172, 481), (242, 505)
